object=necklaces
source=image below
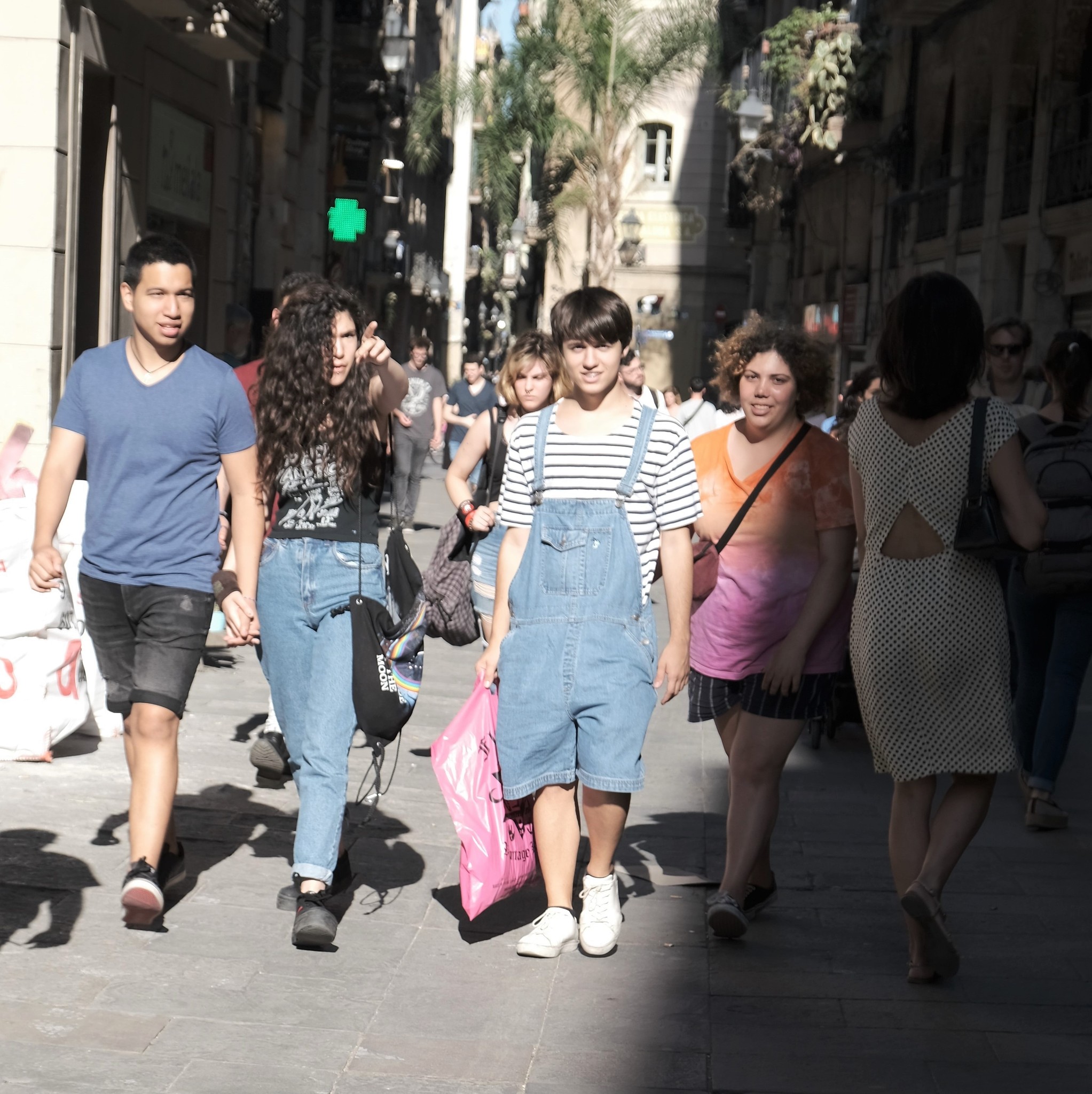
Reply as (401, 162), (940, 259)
(129, 332), (183, 373)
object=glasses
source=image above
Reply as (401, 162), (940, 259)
(987, 344), (1025, 358)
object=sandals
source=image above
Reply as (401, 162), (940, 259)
(901, 878), (961, 986)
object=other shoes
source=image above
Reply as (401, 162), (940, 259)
(250, 731), (291, 787)
(704, 868), (780, 937)
(1025, 795), (1068, 830)
(386, 517), (416, 532)
(120, 840), (188, 929)
(1018, 763), (1037, 799)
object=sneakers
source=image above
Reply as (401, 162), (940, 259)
(276, 843), (353, 913)
(291, 871), (338, 947)
(578, 865), (622, 957)
(516, 906), (578, 959)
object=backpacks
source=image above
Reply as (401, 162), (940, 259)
(1016, 412), (1092, 597)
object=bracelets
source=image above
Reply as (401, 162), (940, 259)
(219, 509), (229, 517)
(211, 570), (242, 612)
(457, 498), (483, 532)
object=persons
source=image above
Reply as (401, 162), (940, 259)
(219, 278), (409, 951)
(689, 312), (850, 946)
(27, 233), (1091, 827)
(474, 287), (705, 960)
(27, 240), (262, 933)
(846, 269), (1044, 982)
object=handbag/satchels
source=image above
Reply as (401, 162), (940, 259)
(420, 508), (481, 646)
(430, 670), (542, 921)
(690, 541), (719, 602)
(349, 413), (427, 804)
(952, 395), (1021, 553)
